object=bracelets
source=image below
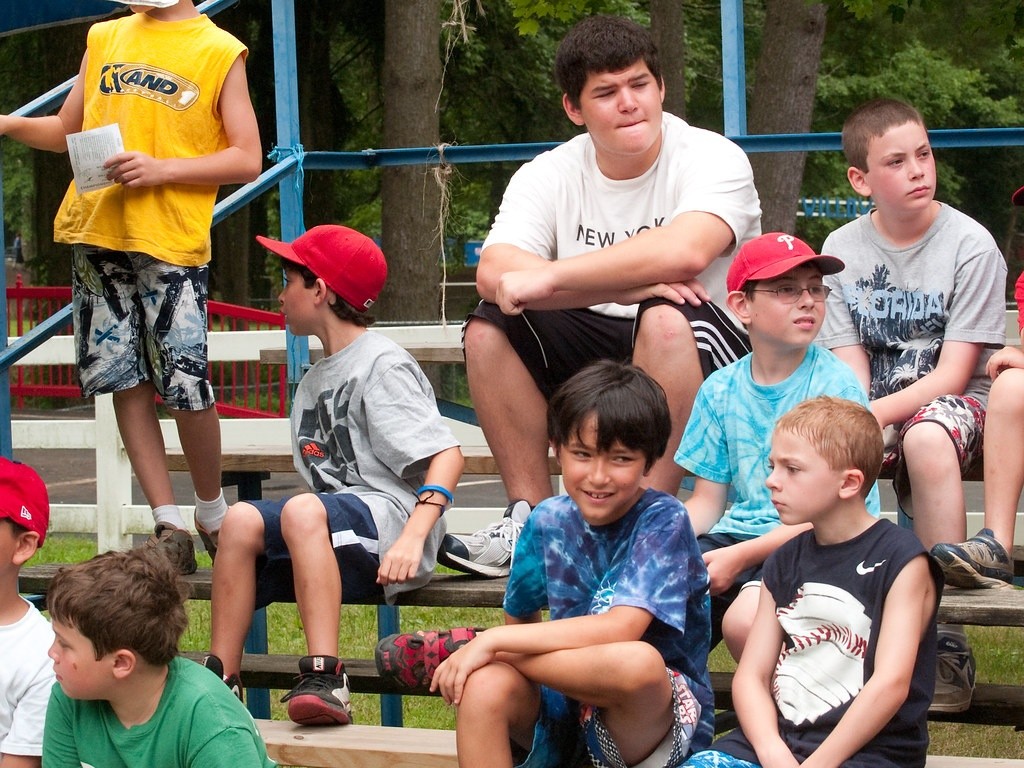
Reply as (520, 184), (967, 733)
(412, 485), (454, 517)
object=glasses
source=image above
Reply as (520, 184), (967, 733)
(743, 282), (833, 305)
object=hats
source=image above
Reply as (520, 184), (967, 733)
(254, 225), (386, 311)
(0, 456), (49, 548)
(727, 232), (845, 292)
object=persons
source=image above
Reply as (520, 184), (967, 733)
(42, 549), (277, 768)
(201, 225), (464, 724)
(375, 360), (716, 768)
(822, 99), (1006, 711)
(436, 16), (763, 578)
(674, 233), (880, 650)
(0, 457), (57, 768)
(678, 396), (944, 768)
(0, 0), (263, 572)
(930, 271), (1024, 589)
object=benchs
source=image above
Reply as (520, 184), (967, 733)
(14, 337), (1024, 768)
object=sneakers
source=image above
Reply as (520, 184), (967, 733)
(279, 655), (353, 724)
(202, 655), (242, 703)
(437, 499), (532, 577)
(145, 521), (197, 575)
(929, 526), (1012, 591)
(927, 631), (977, 712)
(193, 507), (227, 563)
(374, 627), (490, 688)
